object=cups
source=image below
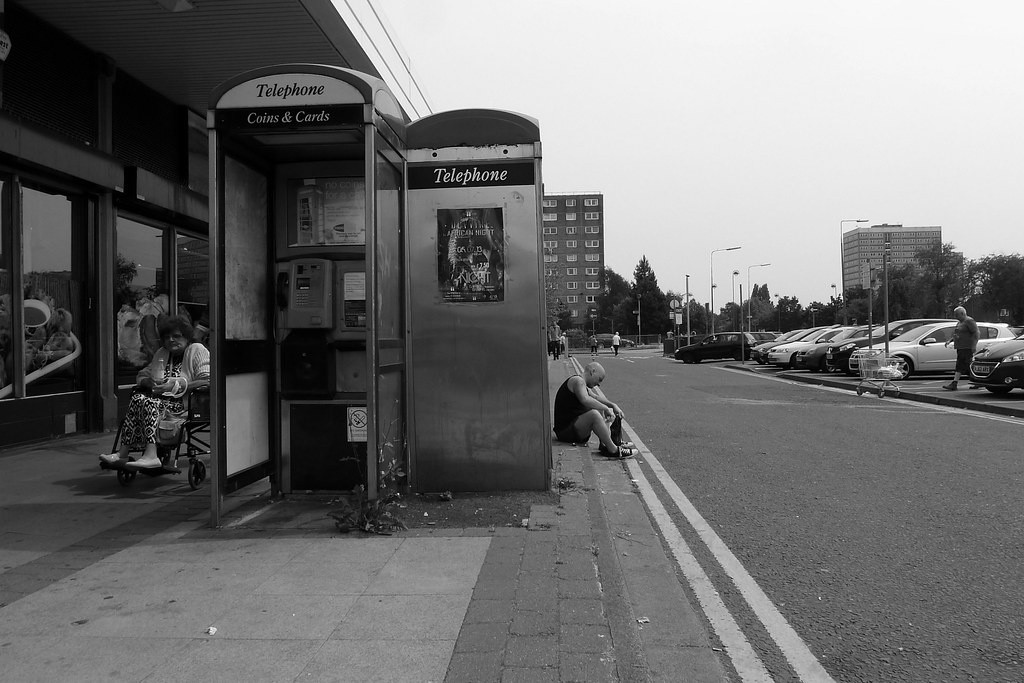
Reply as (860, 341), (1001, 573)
(152, 359), (164, 381)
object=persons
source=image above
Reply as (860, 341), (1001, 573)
(591, 336), (599, 356)
(942, 306), (981, 390)
(0, 288), (72, 390)
(117, 287), (210, 368)
(691, 329), (696, 335)
(98, 313), (211, 469)
(547, 325), (566, 360)
(666, 329), (674, 338)
(613, 332), (620, 356)
(553, 361), (639, 459)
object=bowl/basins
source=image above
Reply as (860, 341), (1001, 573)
(24, 299), (50, 327)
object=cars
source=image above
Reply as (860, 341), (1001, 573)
(750, 328), (809, 365)
(746, 331), (783, 346)
(849, 321), (1024, 381)
(588, 333), (634, 350)
(766, 326), (860, 373)
(968, 331), (1024, 395)
(674, 332), (758, 364)
(795, 326), (885, 374)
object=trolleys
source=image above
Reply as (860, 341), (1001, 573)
(856, 349), (906, 399)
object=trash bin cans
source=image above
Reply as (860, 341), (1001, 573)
(664, 339), (675, 356)
(675, 336), (694, 349)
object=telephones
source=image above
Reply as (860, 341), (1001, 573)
(276, 261), (335, 332)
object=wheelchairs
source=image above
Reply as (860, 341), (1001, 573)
(99, 382), (212, 490)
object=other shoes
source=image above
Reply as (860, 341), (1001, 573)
(969, 384), (980, 391)
(98, 453), (132, 466)
(942, 380), (958, 391)
(125, 457), (162, 469)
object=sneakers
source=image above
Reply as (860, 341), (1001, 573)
(600, 438), (633, 450)
(608, 444), (638, 461)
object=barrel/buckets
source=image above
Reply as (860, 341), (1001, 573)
(664, 336), (694, 353)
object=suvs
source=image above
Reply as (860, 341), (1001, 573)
(825, 318), (960, 376)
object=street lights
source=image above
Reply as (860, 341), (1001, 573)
(840, 219), (870, 324)
(710, 246), (742, 334)
(747, 263), (771, 332)
